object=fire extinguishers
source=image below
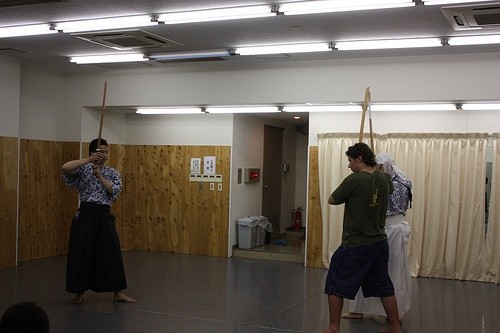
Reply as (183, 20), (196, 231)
(294, 207), (303, 230)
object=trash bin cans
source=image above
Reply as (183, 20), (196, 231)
(237, 214), (268, 249)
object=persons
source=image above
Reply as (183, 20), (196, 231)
(61, 138), (136, 304)
(0, 301), (50, 333)
(324, 143), (414, 333)
